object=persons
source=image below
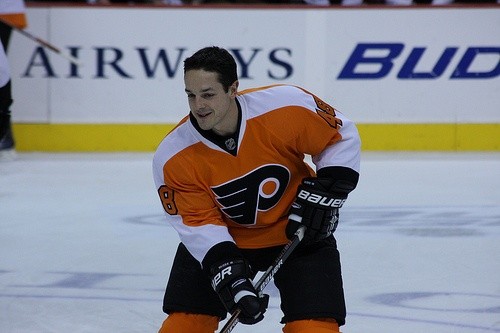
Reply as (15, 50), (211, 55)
(0, 1), (27, 151)
(151, 45), (361, 333)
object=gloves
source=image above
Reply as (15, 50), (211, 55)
(285, 176), (356, 247)
(206, 257), (270, 325)
